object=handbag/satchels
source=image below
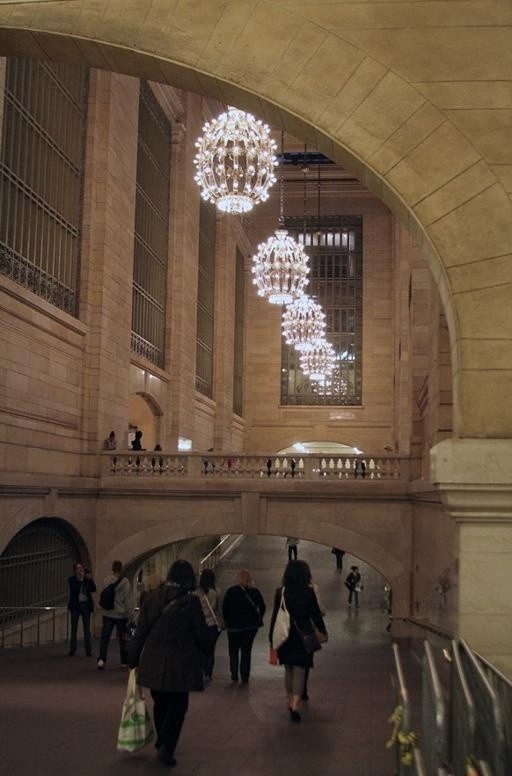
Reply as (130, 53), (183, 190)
(300, 630), (322, 655)
(213, 589), (226, 630)
(115, 665), (156, 754)
(269, 584), (293, 652)
(344, 580), (355, 591)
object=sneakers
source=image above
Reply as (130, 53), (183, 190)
(96, 658), (105, 669)
(120, 662), (127, 669)
(154, 737), (178, 768)
(229, 672), (250, 683)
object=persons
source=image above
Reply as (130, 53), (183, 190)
(268, 561), (328, 725)
(139, 573), (161, 608)
(127, 559), (214, 768)
(104, 429), (118, 465)
(130, 428), (147, 465)
(331, 547), (346, 573)
(96, 560), (137, 671)
(344, 566), (363, 609)
(222, 568), (266, 686)
(67, 562), (98, 658)
(285, 538), (299, 562)
(302, 564), (327, 702)
(194, 566), (226, 683)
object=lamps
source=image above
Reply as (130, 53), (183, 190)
(192, 105), (281, 217)
(250, 128), (347, 402)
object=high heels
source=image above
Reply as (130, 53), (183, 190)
(287, 705), (302, 723)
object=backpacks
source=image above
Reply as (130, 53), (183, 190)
(99, 575), (127, 612)
